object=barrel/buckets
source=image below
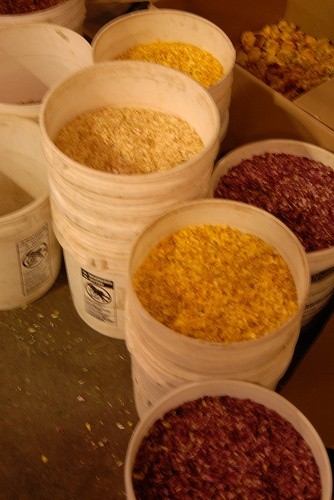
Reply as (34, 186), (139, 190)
(125, 198), (311, 423)
(0, 0), (87, 37)
(209, 139), (333, 326)
(0, 23), (93, 122)
(91, 8), (236, 141)
(39, 60), (220, 340)
(0, 114), (61, 311)
(124, 379), (333, 500)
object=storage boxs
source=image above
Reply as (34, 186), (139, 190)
(148, 0), (334, 152)
